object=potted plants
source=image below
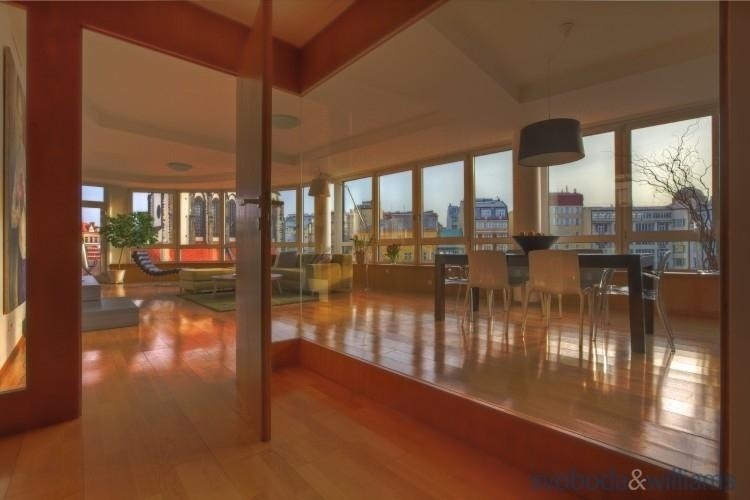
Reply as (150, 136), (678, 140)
(350, 234), (374, 264)
(99, 209), (161, 284)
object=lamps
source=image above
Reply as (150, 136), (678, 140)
(518, 20), (585, 167)
(306, 171), (332, 198)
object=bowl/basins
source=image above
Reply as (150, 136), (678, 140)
(513, 235), (560, 255)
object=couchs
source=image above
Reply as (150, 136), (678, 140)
(178, 251), (353, 299)
(82, 274), (139, 332)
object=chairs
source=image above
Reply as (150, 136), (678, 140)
(433, 248), (677, 353)
(132, 251), (183, 284)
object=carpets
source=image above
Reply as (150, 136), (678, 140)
(183, 287), (318, 313)
